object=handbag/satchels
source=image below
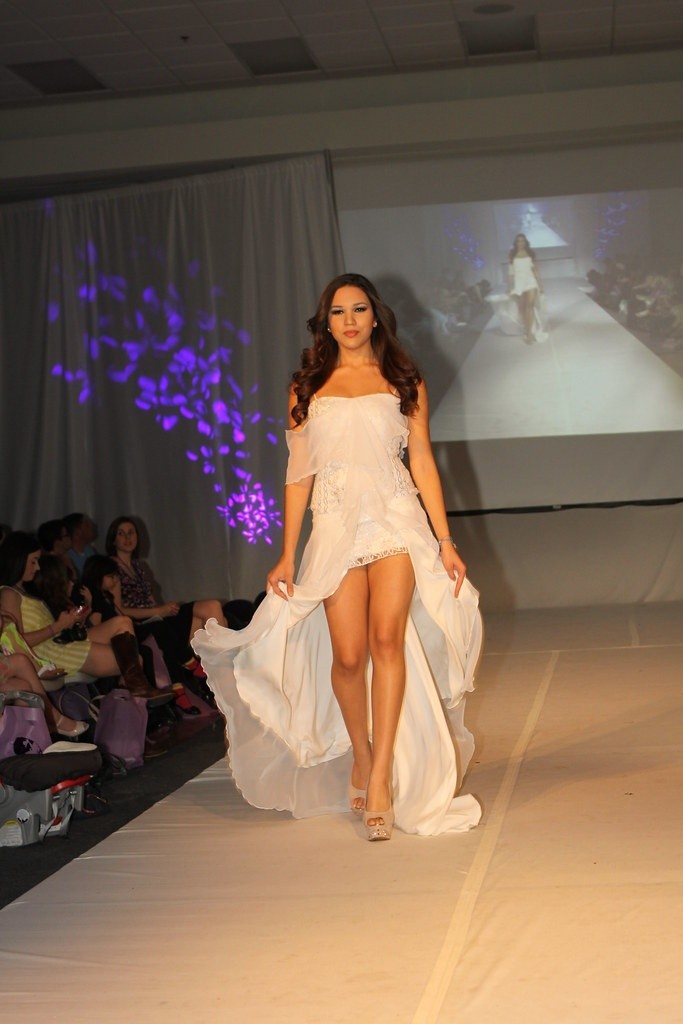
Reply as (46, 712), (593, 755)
(0, 705), (52, 761)
(92, 689), (148, 771)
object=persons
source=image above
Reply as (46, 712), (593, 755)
(382, 265), (494, 351)
(584, 257), (683, 352)
(189, 273), (483, 840)
(485, 234), (546, 345)
(0, 513), (267, 779)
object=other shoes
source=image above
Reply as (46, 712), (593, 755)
(47, 713), (89, 738)
(195, 674), (214, 699)
(173, 693), (200, 715)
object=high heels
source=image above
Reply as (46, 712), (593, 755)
(349, 740), (372, 813)
(362, 794), (393, 840)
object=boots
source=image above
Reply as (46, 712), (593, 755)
(109, 631), (174, 706)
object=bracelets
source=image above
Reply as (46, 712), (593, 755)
(50, 624), (56, 637)
(438, 538), (457, 547)
